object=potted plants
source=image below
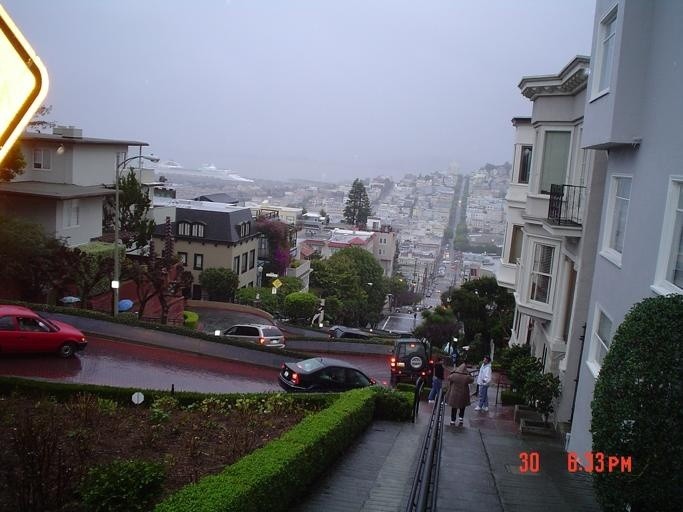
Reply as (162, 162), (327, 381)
(508, 354), (561, 437)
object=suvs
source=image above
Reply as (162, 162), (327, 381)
(386, 337), (434, 389)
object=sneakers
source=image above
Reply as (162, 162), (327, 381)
(474, 405), (490, 413)
(427, 400), (448, 407)
(449, 418), (463, 426)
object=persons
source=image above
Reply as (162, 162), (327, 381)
(445, 362), (474, 426)
(471, 355), (492, 412)
(426, 357), (447, 407)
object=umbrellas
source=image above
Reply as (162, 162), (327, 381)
(58, 296), (80, 304)
(116, 298), (135, 312)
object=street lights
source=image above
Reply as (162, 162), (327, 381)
(112, 150), (160, 315)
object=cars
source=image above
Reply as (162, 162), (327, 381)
(277, 357), (381, 394)
(0, 305), (87, 360)
(208, 321), (285, 349)
